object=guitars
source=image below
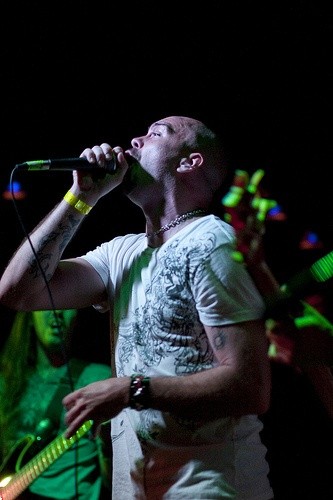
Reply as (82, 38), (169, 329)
(0, 411), (101, 500)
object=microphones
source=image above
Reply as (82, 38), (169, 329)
(16, 153), (134, 174)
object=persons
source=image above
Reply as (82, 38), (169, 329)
(0, 117), (278, 500)
(0, 308), (115, 500)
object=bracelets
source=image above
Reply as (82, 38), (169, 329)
(129, 374), (152, 412)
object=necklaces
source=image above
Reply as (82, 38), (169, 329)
(146, 207), (209, 239)
